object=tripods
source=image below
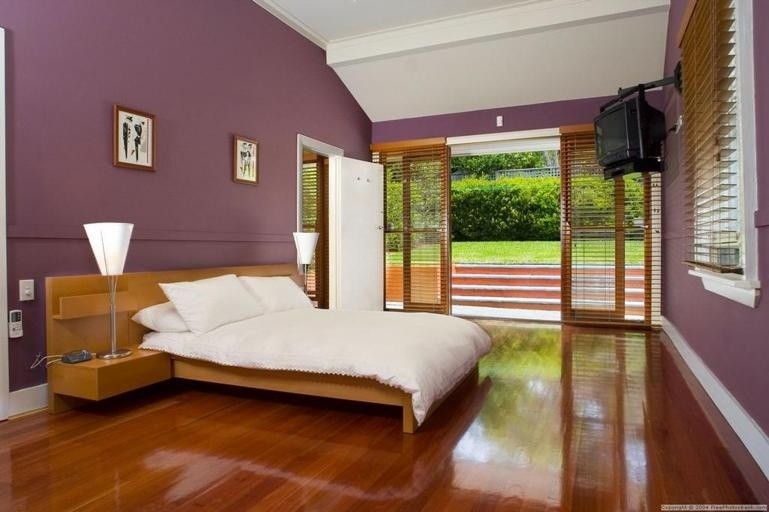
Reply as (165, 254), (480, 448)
(594, 97), (666, 166)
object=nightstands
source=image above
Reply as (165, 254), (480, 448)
(44, 273), (173, 415)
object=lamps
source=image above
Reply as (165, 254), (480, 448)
(81, 222), (135, 361)
(292, 232), (320, 295)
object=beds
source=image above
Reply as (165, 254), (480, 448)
(129, 263), (481, 434)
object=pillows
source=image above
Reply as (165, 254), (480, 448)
(130, 273), (315, 336)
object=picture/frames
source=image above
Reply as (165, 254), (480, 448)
(232, 133), (260, 187)
(112, 101), (158, 172)
